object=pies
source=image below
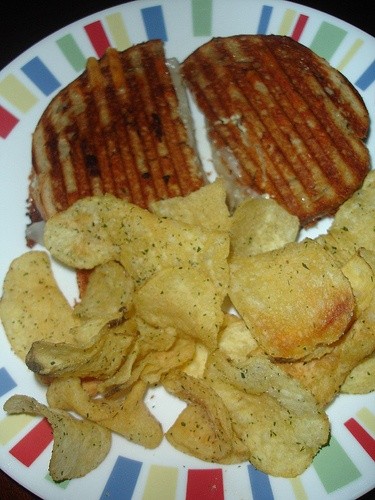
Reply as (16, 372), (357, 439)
(25, 40), (209, 248)
(179, 35), (372, 229)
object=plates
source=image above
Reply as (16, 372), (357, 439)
(0, 0), (375, 500)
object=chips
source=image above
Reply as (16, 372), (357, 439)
(1, 166), (375, 484)
